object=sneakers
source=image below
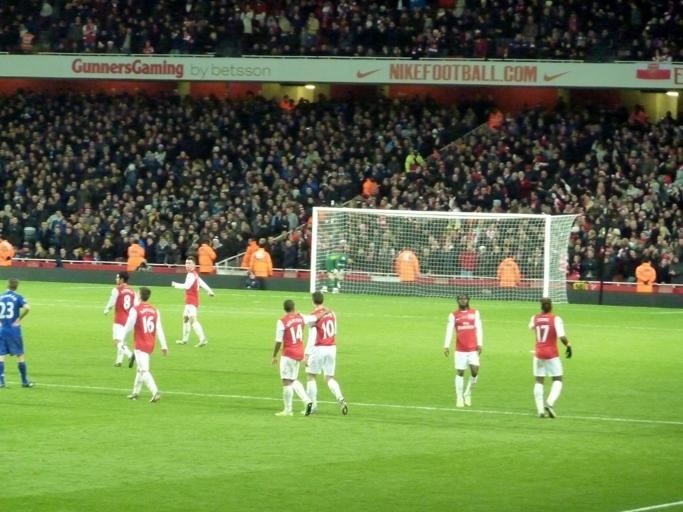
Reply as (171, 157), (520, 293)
(195, 340), (207, 347)
(176, 341), (187, 345)
(540, 404), (556, 417)
(22, 382), (33, 387)
(464, 393), (471, 406)
(275, 397), (348, 415)
(456, 398), (464, 407)
(127, 394), (137, 399)
(129, 353), (135, 368)
(151, 392), (160, 402)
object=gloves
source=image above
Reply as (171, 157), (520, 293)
(566, 343), (571, 358)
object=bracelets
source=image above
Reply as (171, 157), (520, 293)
(566, 342), (571, 347)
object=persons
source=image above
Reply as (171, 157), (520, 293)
(0, 277), (36, 389)
(171, 255), (215, 347)
(529, 297), (572, 417)
(117, 287), (168, 403)
(2, 0), (682, 296)
(443, 294), (483, 408)
(303, 292), (349, 416)
(272, 299), (332, 417)
(103, 270), (136, 368)
